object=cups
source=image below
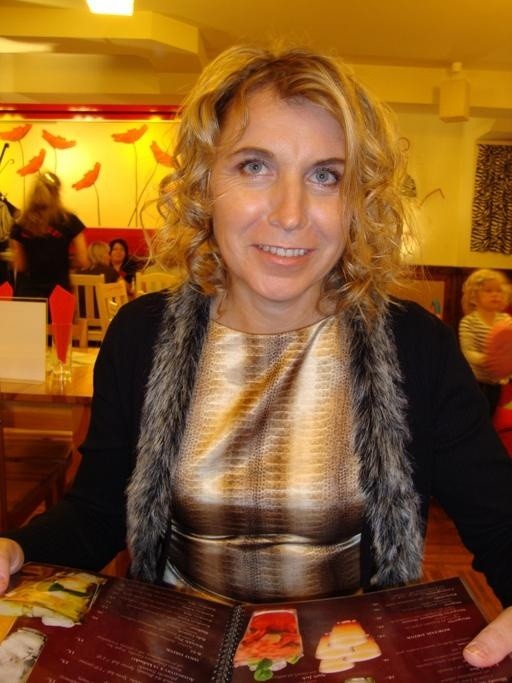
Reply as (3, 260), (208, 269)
(1, 625), (49, 682)
(232, 608), (304, 668)
(2, 567), (104, 621)
(51, 321), (75, 385)
(315, 617), (382, 673)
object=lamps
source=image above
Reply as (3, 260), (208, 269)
(437, 60), (472, 123)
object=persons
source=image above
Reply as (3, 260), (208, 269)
(454, 269), (512, 418)
(0, 171), (139, 346)
(1, 43), (512, 669)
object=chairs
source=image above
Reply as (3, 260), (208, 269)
(46, 272), (185, 354)
